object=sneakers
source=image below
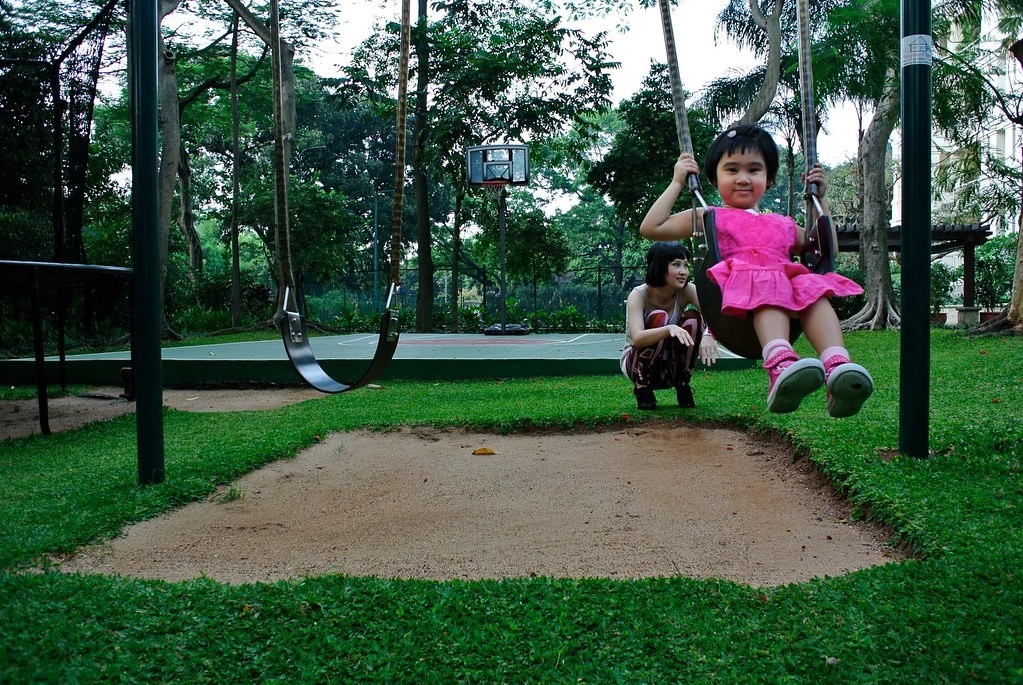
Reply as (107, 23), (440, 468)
(759, 349), (826, 413)
(822, 353), (874, 418)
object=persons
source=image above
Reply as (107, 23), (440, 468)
(638, 125), (874, 418)
(618, 242), (721, 410)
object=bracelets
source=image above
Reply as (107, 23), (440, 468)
(701, 333), (714, 337)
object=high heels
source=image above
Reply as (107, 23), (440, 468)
(633, 384), (659, 410)
(674, 383), (696, 409)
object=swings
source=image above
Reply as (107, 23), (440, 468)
(267, 0), (411, 393)
(659, 0), (840, 359)
